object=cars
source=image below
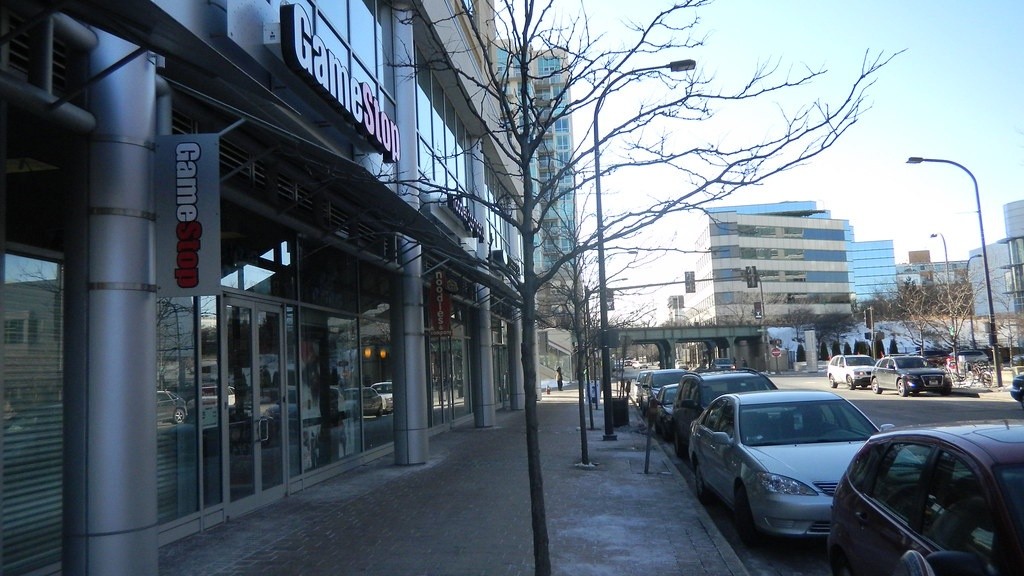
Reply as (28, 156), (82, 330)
(674, 359), (689, 370)
(654, 383), (682, 441)
(688, 389), (895, 544)
(624, 360), (648, 370)
(1012, 353), (1024, 365)
(632, 368), (686, 418)
(957, 350), (989, 369)
(712, 357), (736, 370)
(909, 350), (949, 367)
(827, 418), (1024, 576)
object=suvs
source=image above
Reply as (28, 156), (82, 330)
(826, 354), (877, 390)
(870, 354), (952, 397)
(673, 367), (803, 460)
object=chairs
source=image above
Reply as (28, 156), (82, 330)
(948, 473), (977, 504)
(742, 411), (777, 444)
(885, 484), (918, 519)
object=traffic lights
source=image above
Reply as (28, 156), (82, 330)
(863, 309), (871, 329)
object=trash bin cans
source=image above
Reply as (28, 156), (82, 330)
(612, 396), (629, 427)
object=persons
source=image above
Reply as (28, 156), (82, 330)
(741, 356), (747, 367)
(555, 365), (563, 391)
(319, 360), (355, 450)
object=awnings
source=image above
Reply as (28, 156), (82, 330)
(426, 248), (550, 323)
(155, 73), (478, 253)
(0, 0), (301, 129)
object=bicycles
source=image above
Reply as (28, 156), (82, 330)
(942, 360), (993, 388)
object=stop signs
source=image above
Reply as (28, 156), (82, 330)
(772, 348), (781, 357)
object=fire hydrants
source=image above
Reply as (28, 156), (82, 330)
(545, 385), (551, 394)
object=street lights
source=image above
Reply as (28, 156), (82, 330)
(967, 254), (983, 349)
(931, 233), (959, 374)
(730, 269), (771, 374)
(594, 58), (695, 442)
(906, 156), (1004, 387)
